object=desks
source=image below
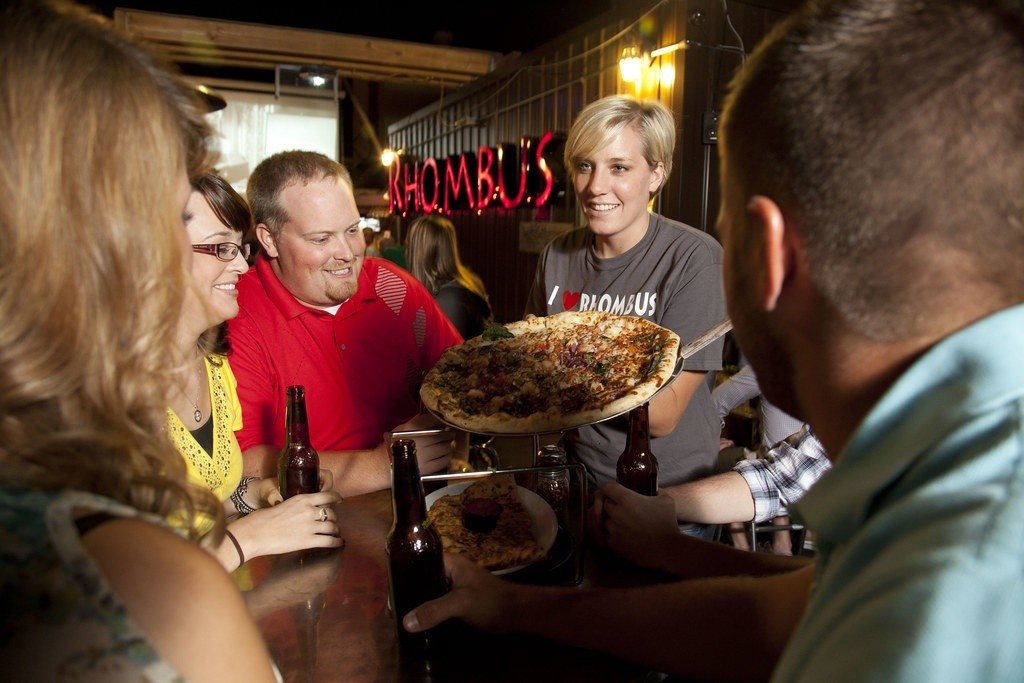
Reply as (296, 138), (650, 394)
(228, 482), (680, 682)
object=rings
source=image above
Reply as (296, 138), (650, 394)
(319, 506), (328, 521)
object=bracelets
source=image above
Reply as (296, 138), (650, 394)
(225, 529), (244, 566)
(231, 476), (259, 515)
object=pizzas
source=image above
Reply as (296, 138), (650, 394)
(430, 466), (542, 570)
(420, 309), (681, 432)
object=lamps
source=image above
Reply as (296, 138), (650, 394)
(300, 66), (335, 88)
(619, 43), (650, 81)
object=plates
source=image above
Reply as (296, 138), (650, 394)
(424, 479), (557, 576)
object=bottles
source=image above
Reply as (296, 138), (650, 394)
(534, 444), (570, 526)
(276, 385), (324, 502)
(285, 550), (326, 675)
(388, 438), (469, 683)
(616, 402), (659, 496)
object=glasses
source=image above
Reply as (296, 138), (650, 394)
(193, 240), (251, 262)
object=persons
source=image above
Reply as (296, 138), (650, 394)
(131, 171), (343, 574)
(364, 227), (408, 271)
(403, 215), (492, 341)
(226, 152), (465, 497)
(0, 4), (285, 683)
(229, 553), (342, 624)
(521, 94), (730, 587)
(403, 0), (1024, 683)
(588, 368), (832, 580)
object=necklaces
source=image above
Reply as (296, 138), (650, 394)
(182, 364), (202, 422)
